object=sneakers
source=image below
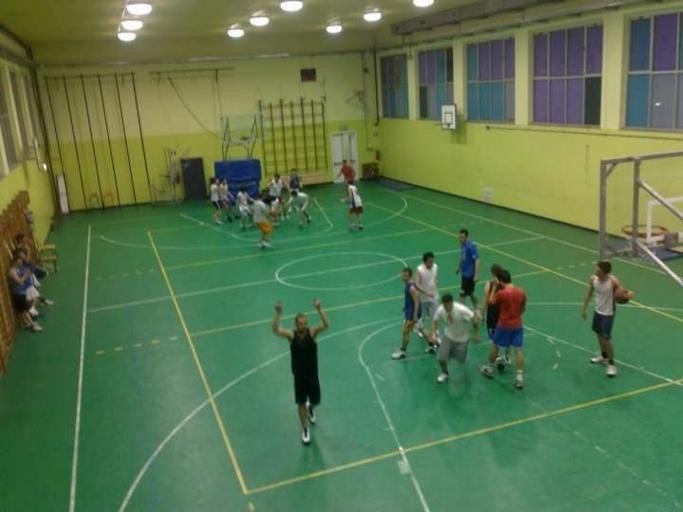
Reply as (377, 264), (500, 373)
(306, 402), (314, 423)
(215, 218), (224, 224)
(42, 300), (53, 306)
(481, 365), (495, 377)
(228, 214), (254, 230)
(350, 224), (362, 231)
(392, 351), (407, 359)
(303, 428), (310, 442)
(34, 312), (44, 318)
(496, 354), (511, 365)
(606, 366), (616, 376)
(425, 339), (441, 353)
(437, 373), (449, 383)
(591, 356), (607, 364)
(516, 380), (523, 388)
(418, 332), (424, 338)
(26, 321), (42, 332)
(298, 216), (311, 228)
(258, 240), (271, 249)
(272, 208), (291, 227)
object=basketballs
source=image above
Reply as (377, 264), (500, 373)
(614, 288), (629, 304)
(269, 206), (278, 215)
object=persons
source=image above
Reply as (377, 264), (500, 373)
(481, 264), (512, 365)
(455, 229), (480, 311)
(271, 298), (329, 446)
(336, 159), (367, 233)
(414, 251), (443, 346)
(582, 259), (634, 377)
(392, 267), (435, 358)
(205, 169), (311, 249)
(430, 294), (478, 384)
(482, 271), (527, 390)
(8, 232), (54, 333)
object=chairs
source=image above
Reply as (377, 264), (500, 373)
(37, 248), (58, 273)
(35, 237), (57, 255)
